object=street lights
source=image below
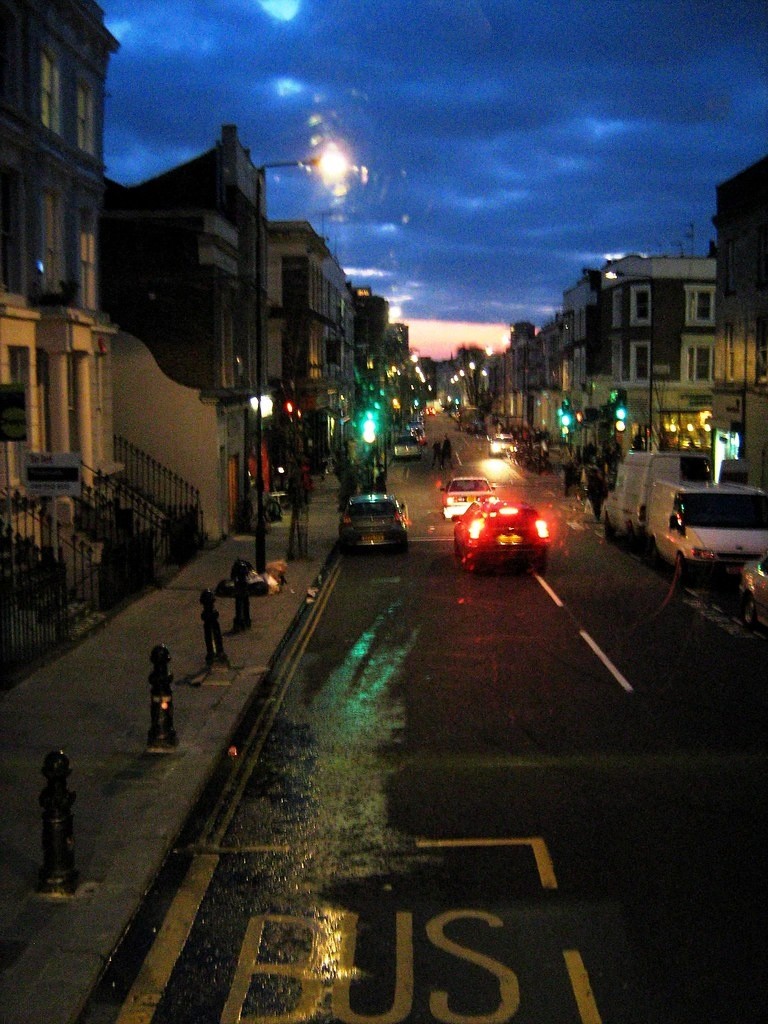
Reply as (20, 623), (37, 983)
(247, 145), (346, 575)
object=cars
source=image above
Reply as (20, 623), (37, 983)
(438, 477), (496, 521)
(450, 499), (553, 576)
(487, 432), (515, 458)
(394, 435), (425, 462)
(738, 549), (768, 636)
(339, 491), (410, 556)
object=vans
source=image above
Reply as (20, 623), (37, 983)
(602, 450), (714, 548)
(646, 479), (768, 582)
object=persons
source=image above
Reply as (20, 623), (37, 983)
(361, 460), (387, 494)
(430, 433), (453, 471)
(496, 423), (647, 524)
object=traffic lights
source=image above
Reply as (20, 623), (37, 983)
(615, 388), (628, 433)
(560, 401), (574, 439)
(575, 408), (584, 423)
